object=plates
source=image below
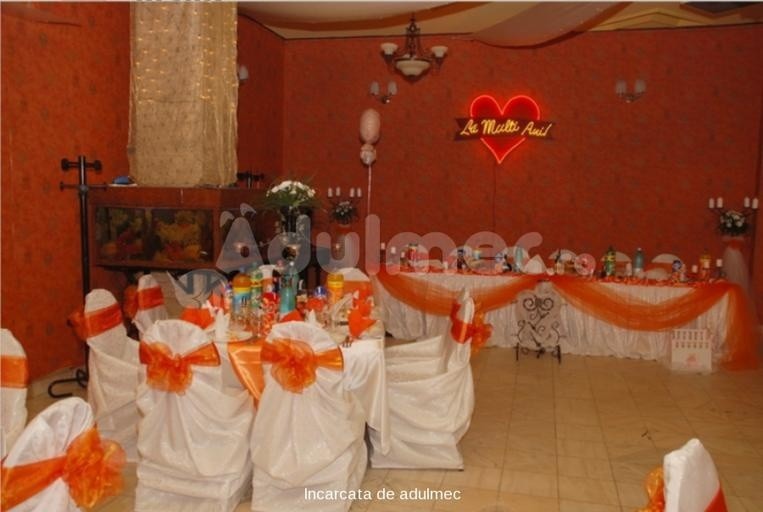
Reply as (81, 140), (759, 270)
(206, 329), (252, 342)
(574, 253), (596, 276)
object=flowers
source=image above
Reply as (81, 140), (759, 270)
(327, 200), (360, 223)
(265, 178), (317, 215)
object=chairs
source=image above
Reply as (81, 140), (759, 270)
(510, 285), (565, 363)
(0, 268), (476, 510)
(657, 433), (725, 511)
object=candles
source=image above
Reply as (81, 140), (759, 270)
(381, 242), (405, 258)
(708, 196), (758, 210)
(326, 186), (362, 197)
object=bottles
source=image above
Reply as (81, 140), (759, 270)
(515, 246), (523, 271)
(408, 244), (419, 269)
(554, 247), (564, 275)
(604, 245), (615, 277)
(671, 250), (711, 285)
(231, 261), (345, 327)
(633, 246), (644, 277)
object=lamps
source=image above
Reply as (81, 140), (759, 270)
(372, 80), (399, 105)
(377, 9), (450, 78)
(616, 80), (647, 103)
